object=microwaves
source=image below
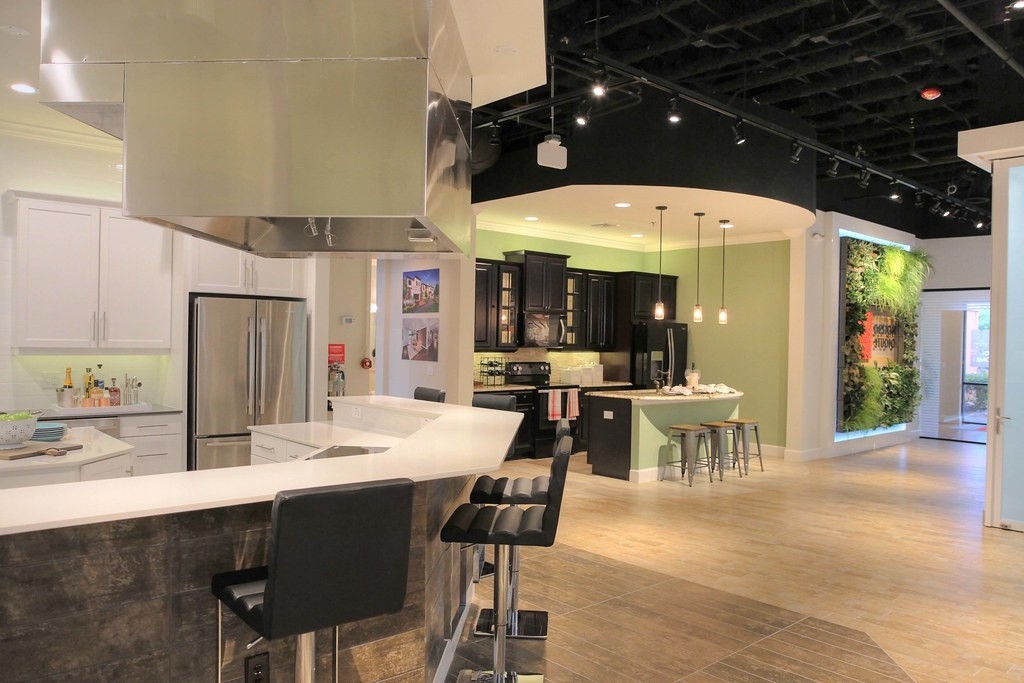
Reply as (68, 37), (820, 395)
(518, 313), (568, 348)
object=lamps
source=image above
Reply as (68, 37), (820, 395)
(667, 96), (686, 123)
(858, 168), (870, 188)
(731, 118), (746, 144)
(718, 220), (730, 324)
(693, 212), (705, 322)
(824, 156), (840, 177)
(913, 192), (983, 228)
(654, 206), (667, 320)
(888, 183), (900, 199)
(574, 97), (593, 126)
(592, 65), (611, 96)
(788, 142), (802, 164)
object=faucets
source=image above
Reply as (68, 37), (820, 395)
(656, 369), (670, 386)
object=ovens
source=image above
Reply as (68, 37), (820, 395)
(536, 388), (579, 460)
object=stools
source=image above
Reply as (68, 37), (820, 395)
(441, 436), (573, 683)
(660, 419), (763, 487)
(469, 418), (571, 638)
(210, 478), (414, 683)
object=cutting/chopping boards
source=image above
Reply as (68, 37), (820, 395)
(0, 443), (84, 461)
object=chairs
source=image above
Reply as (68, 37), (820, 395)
(472, 394), (517, 458)
(414, 386), (446, 404)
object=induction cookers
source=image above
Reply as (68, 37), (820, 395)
(505, 362), (579, 390)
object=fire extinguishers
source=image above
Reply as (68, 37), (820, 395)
(328, 362), (345, 411)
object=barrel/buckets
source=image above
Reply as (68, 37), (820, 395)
(57, 387), (80, 408)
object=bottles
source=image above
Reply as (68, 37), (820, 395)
(63, 362), (122, 408)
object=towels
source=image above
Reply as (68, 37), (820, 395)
(566, 388), (579, 421)
(548, 389), (561, 421)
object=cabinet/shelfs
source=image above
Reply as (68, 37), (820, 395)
(473, 248), (679, 353)
(6, 196), (307, 353)
(59, 413), (186, 476)
(514, 391), (537, 458)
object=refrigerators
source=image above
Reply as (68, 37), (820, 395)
(189, 296), (307, 470)
(635, 321), (688, 389)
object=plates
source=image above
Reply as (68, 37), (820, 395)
(30, 423), (69, 441)
